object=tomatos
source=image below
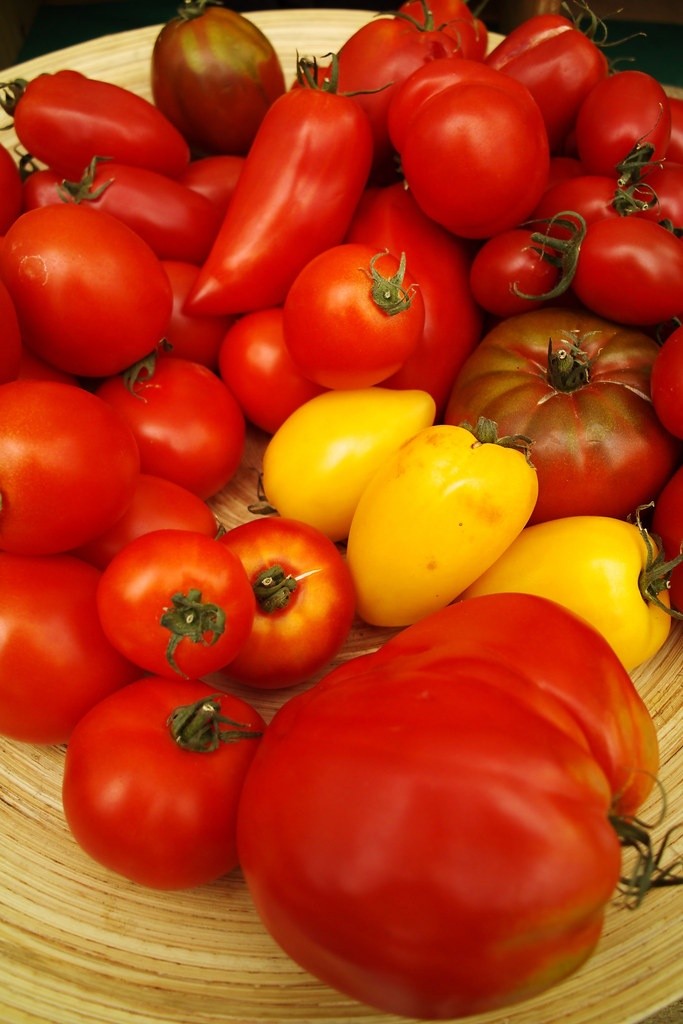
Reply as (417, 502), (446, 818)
(1, 0), (680, 1022)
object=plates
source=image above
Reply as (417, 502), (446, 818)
(6, 8), (680, 1022)
